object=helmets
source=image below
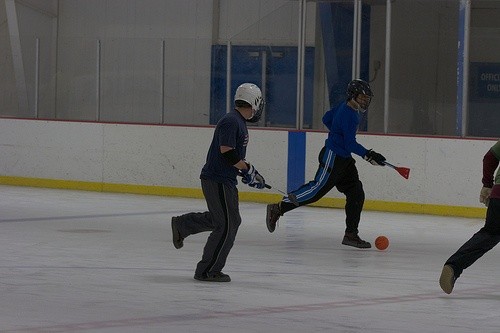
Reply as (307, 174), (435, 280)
(234, 82), (264, 122)
(347, 78), (374, 111)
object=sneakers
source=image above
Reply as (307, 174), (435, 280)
(266, 202), (280, 232)
(342, 234), (371, 249)
(171, 215), (184, 249)
(193, 270), (231, 282)
(440, 264), (458, 293)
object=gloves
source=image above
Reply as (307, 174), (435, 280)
(241, 160), (256, 184)
(249, 170), (265, 189)
(364, 147), (387, 166)
(480, 186), (492, 207)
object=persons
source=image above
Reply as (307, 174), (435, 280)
(171, 83), (266, 283)
(266, 78), (387, 248)
(439, 136), (500, 294)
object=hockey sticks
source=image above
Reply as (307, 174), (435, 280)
(382, 161), (411, 180)
(237, 171), (300, 206)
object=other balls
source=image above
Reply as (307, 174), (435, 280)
(374, 235), (390, 251)
